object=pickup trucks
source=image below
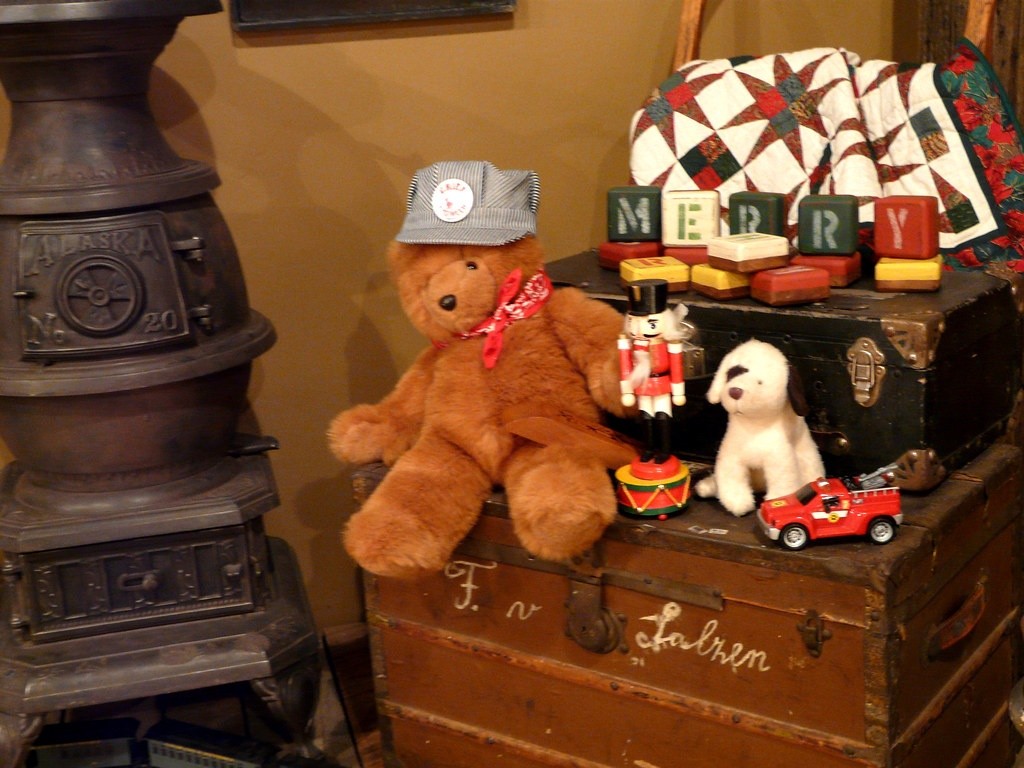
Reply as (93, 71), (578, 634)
(757, 463), (903, 551)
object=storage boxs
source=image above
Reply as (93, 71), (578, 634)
(546, 248), (1024, 495)
(343, 444), (1024, 768)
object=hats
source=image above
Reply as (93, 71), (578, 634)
(395, 161), (540, 246)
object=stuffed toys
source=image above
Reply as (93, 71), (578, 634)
(323, 158), (629, 586)
(708, 338), (826, 513)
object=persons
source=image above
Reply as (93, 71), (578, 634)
(613, 280), (690, 419)
